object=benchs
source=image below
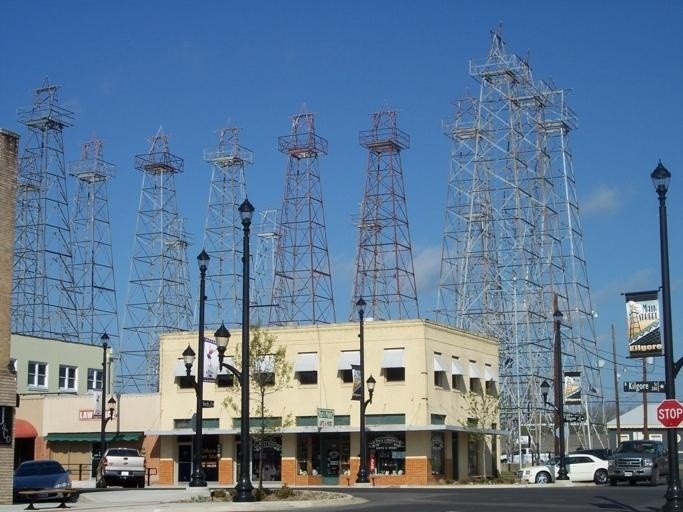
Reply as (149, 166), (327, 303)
(18, 489), (77, 510)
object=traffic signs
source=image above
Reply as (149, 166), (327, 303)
(623, 381), (667, 393)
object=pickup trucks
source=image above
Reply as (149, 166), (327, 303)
(608, 440), (670, 487)
(95, 446), (147, 489)
(500, 439), (551, 465)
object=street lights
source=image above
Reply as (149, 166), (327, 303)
(355, 294), (376, 484)
(539, 305), (578, 480)
(649, 157), (683, 512)
(100, 331), (117, 457)
(213, 192), (256, 503)
(181, 246), (211, 488)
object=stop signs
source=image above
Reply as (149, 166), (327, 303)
(656, 399), (683, 427)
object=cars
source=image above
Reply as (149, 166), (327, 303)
(517, 453), (610, 485)
(12, 458), (72, 504)
